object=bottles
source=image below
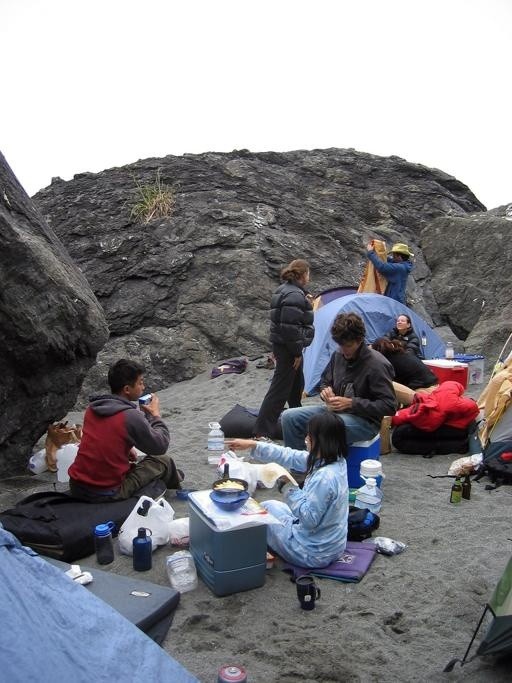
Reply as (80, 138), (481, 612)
(450, 474), (462, 503)
(217, 664), (248, 683)
(354, 478), (383, 518)
(462, 471), (471, 500)
(360, 459), (385, 489)
(132, 527), (153, 572)
(445, 340), (455, 358)
(207, 422), (224, 464)
(93, 520), (116, 565)
(166, 549), (198, 594)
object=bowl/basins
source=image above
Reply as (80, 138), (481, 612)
(212, 478), (249, 493)
(209, 489), (249, 511)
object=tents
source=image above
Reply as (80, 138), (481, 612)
(296, 293), (447, 400)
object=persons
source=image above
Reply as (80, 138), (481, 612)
(368, 240), (414, 307)
(250, 258), (314, 446)
(67, 360), (185, 504)
(222, 411), (350, 569)
(381, 314), (425, 360)
(280, 311), (398, 487)
(368, 338), (438, 408)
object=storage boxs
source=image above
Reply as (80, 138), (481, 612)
(440, 351), (483, 385)
(185, 501), (267, 598)
(424, 358), (467, 391)
(44, 558), (180, 649)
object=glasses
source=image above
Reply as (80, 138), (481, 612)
(304, 435), (310, 444)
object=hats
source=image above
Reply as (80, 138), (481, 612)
(387, 244), (414, 257)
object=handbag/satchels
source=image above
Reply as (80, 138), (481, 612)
(45, 420), (82, 472)
(211, 358), (247, 378)
(346, 505), (380, 541)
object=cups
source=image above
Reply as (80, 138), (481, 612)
(295, 575), (321, 611)
